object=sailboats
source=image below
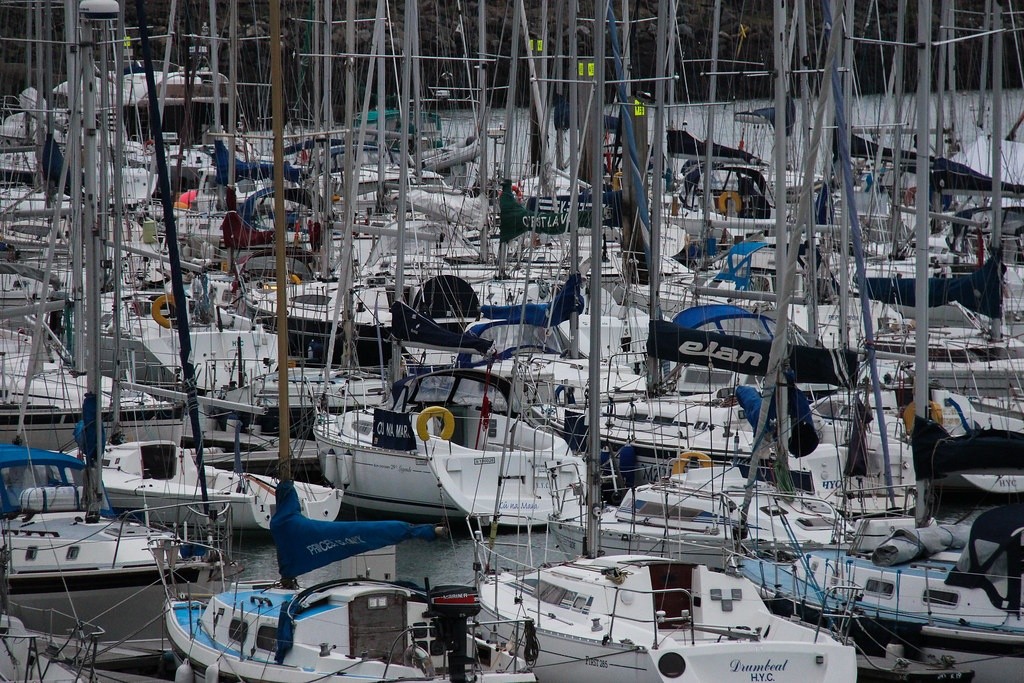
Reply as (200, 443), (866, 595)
(0, 0), (1024, 683)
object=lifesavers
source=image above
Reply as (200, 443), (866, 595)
(416, 405), (455, 442)
(903, 399), (944, 435)
(672, 450), (714, 475)
(152, 295), (177, 328)
(403, 645), (436, 680)
(498, 184), (523, 207)
(718, 191), (742, 214)
(904, 185), (917, 206)
